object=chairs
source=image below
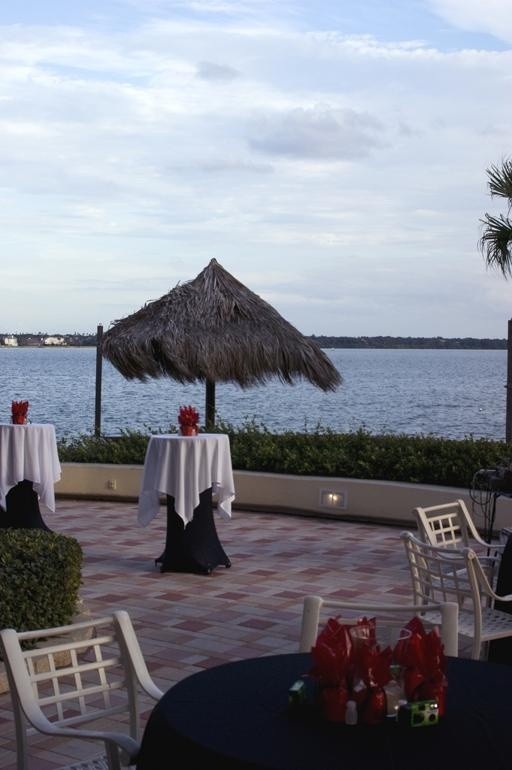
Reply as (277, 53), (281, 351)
(400, 498), (512, 661)
(300, 595), (458, 658)
(0, 610), (164, 769)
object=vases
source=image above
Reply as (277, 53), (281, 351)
(12, 415), (24, 425)
(180, 424), (198, 436)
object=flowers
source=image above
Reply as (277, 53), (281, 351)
(177, 404), (200, 425)
(11, 399), (29, 417)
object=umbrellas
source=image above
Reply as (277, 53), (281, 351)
(96, 257), (345, 421)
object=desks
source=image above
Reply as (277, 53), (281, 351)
(0, 423), (63, 532)
(137, 433), (237, 575)
(135, 650), (512, 770)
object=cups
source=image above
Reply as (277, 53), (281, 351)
(348, 622), (413, 719)
